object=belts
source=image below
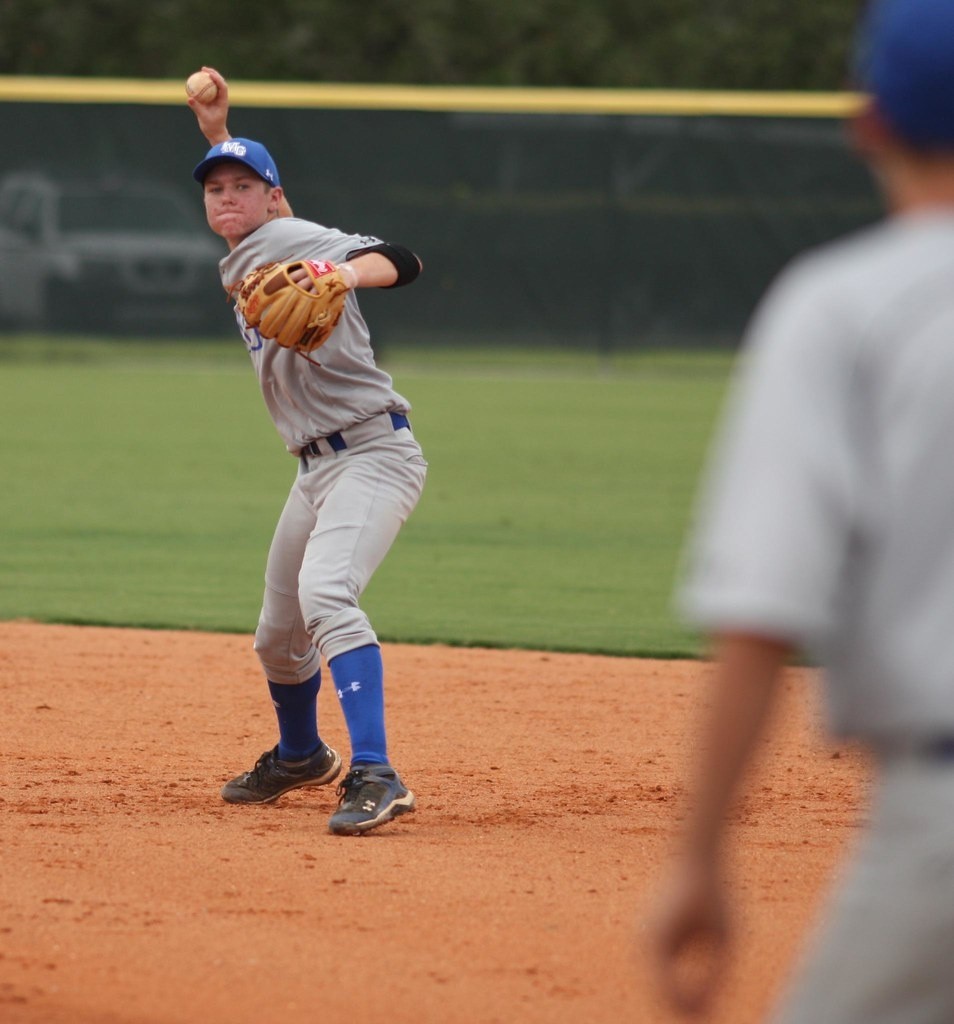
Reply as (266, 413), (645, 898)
(302, 411), (410, 455)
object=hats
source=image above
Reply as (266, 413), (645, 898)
(854, 0), (954, 151)
(193, 137), (280, 190)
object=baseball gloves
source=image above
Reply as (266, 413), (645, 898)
(236, 257), (350, 354)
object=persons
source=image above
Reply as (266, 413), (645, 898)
(642, 1), (954, 1024)
(187, 66), (427, 834)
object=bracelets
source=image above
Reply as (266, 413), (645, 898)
(340, 263), (357, 288)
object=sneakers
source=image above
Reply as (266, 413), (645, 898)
(221, 738), (344, 805)
(329, 765), (414, 836)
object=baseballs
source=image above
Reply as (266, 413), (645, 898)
(185, 72), (219, 103)
